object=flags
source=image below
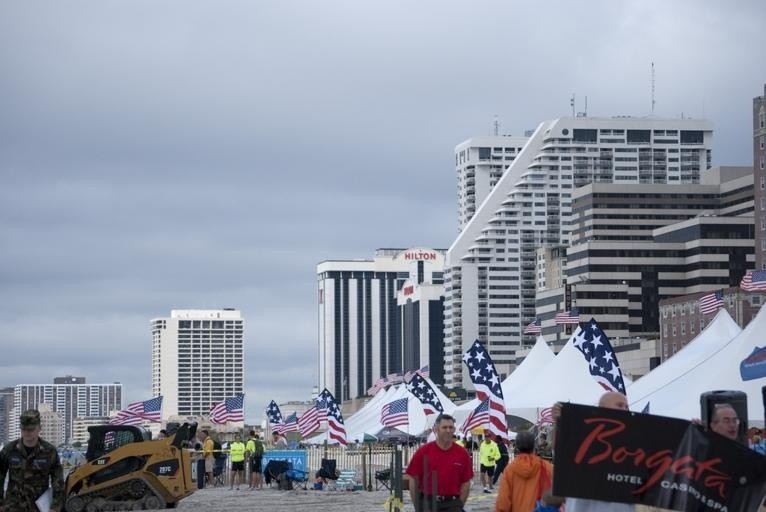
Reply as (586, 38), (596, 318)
(740, 271), (766, 294)
(109, 397), (162, 426)
(523, 319), (541, 334)
(298, 398), (327, 438)
(699, 289), (724, 315)
(277, 412), (297, 435)
(209, 394), (244, 425)
(555, 308), (579, 324)
(367, 365), (429, 397)
(379, 398), (407, 429)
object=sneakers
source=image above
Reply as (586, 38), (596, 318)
(228, 485), (264, 491)
(483, 486), (494, 493)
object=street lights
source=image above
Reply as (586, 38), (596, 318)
(364, 433), (378, 491)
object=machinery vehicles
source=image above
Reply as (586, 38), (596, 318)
(58, 423), (202, 512)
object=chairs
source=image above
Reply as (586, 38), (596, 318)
(212, 454), (227, 487)
(375, 467), (391, 490)
(286, 467), (309, 490)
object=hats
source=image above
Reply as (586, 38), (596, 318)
(18, 409), (41, 431)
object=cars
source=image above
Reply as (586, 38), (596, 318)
(374, 469), (390, 480)
(337, 471), (361, 491)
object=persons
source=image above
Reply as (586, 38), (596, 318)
(453, 387), (766, 511)
(57, 446), (73, 468)
(0, 409), (65, 512)
(159, 430), (289, 490)
(406, 414), (475, 512)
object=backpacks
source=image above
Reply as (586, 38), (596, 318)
(249, 438), (263, 458)
(205, 438), (222, 460)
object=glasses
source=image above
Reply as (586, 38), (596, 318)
(484, 435), (489, 438)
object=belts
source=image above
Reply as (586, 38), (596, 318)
(421, 493), (459, 503)
